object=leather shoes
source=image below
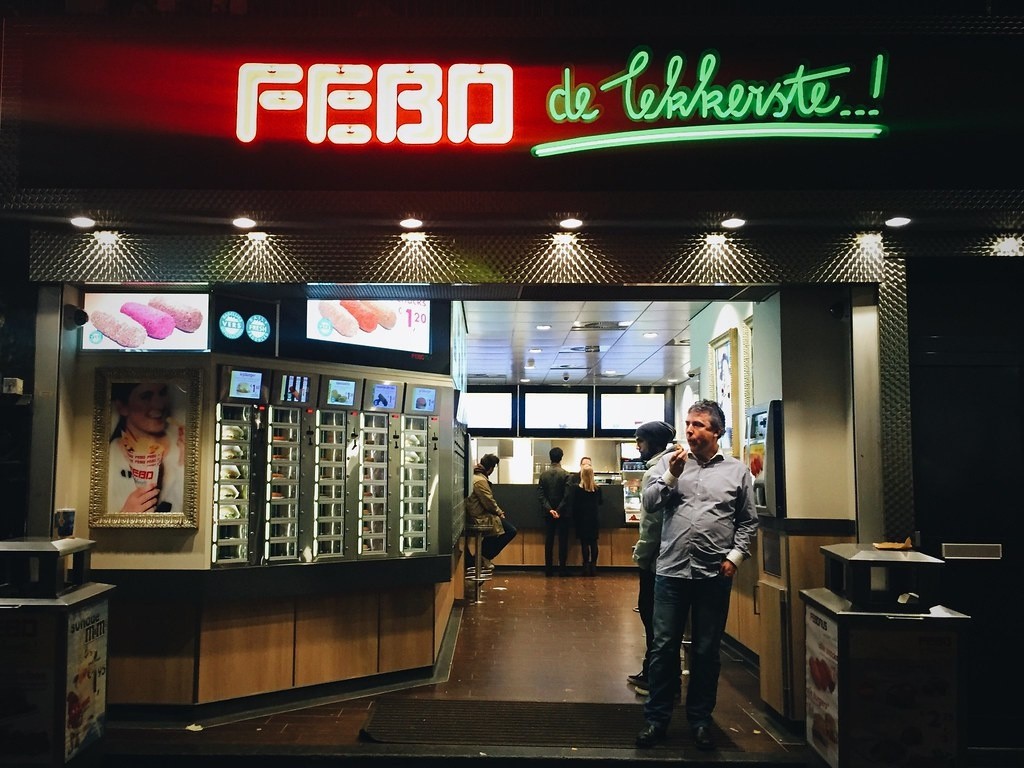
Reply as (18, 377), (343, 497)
(636, 724), (666, 747)
(692, 726), (717, 750)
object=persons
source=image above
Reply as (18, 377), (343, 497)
(537, 448), (602, 577)
(467, 455), (517, 568)
(627, 421), (684, 684)
(635, 399), (759, 747)
(107, 383), (187, 514)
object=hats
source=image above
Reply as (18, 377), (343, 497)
(636, 420), (676, 449)
(550, 448), (563, 461)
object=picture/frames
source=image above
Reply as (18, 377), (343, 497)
(706, 326), (741, 459)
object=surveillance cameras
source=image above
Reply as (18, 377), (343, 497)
(66, 306), (88, 329)
(563, 375), (570, 382)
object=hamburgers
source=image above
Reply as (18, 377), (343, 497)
(220, 426), (245, 519)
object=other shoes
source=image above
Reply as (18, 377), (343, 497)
(559, 568), (569, 577)
(545, 573), (553, 577)
(626, 670), (645, 684)
(472, 553), (495, 569)
(635, 679), (681, 699)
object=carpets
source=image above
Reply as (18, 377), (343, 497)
(359, 694), (745, 752)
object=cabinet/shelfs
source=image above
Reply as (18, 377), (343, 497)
(727, 528), (790, 729)
(104, 564), (456, 731)
(488, 528), (640, 567)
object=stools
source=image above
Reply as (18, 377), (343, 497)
(464, 524), (493, 605)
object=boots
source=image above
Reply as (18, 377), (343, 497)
(583, 561), (597, 577)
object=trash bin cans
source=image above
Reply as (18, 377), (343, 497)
(0, 536), (117, 768)
(798, 543), (972, 768)
